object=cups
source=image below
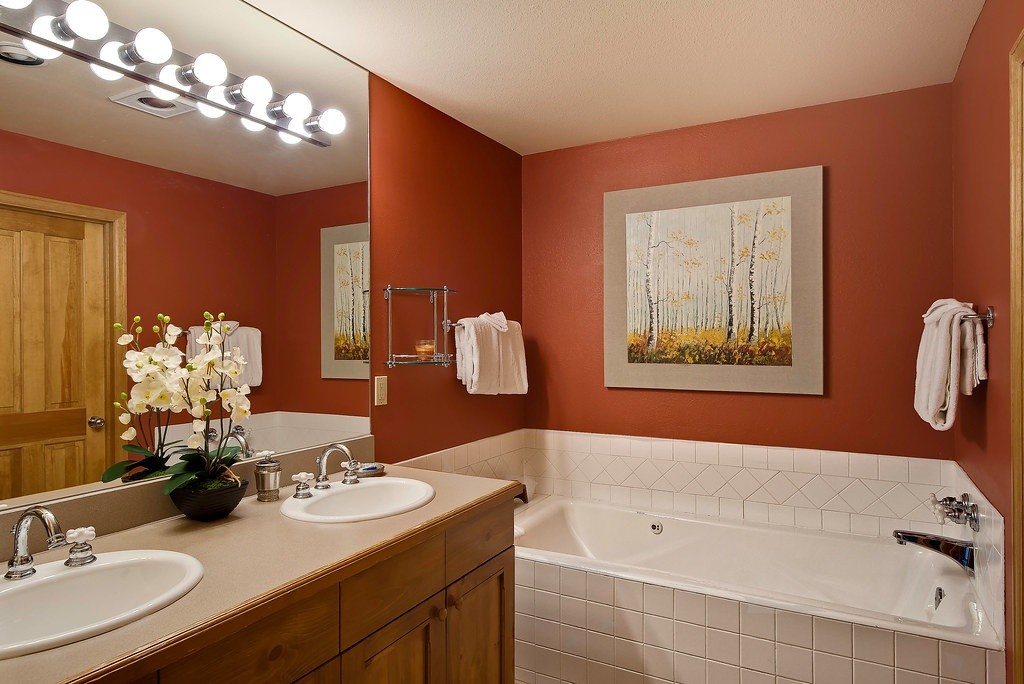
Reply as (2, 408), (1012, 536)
(415, 340), (435, 362)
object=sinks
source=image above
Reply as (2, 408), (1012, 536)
(279, 477), (436, 523)
(1, 549), (205, 661)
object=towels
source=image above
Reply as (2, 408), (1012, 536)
(454, 311), (529, 395)
(185, 319), (263, 391)
(913, 298), (991, 432)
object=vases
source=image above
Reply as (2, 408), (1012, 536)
(169, 476), (249, 519)
(122, 468), (170, 482)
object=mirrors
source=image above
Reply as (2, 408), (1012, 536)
(0, 1), (370, 507)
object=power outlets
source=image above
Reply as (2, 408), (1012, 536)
(375, 376), (387, 405)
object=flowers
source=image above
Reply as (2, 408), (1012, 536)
(102, 314), (189, 483)
(162, 310), (252, 496)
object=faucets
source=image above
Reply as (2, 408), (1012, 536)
(892, 529), (975, 578)
(3, 505), (67, 580)
(314, 443), (358, 489)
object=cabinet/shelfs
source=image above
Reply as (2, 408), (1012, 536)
(88, 500), (515, 684)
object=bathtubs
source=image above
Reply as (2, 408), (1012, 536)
(514, 496), (1006, 682)
